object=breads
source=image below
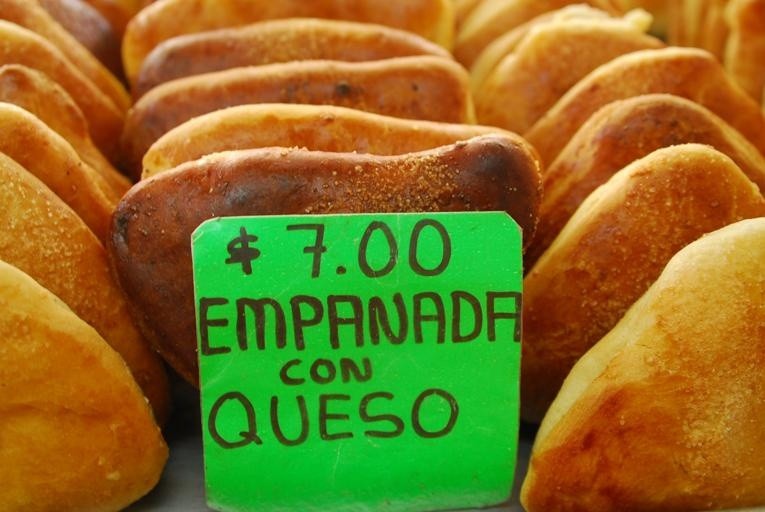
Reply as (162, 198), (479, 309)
(0, 0), (765, 512)
